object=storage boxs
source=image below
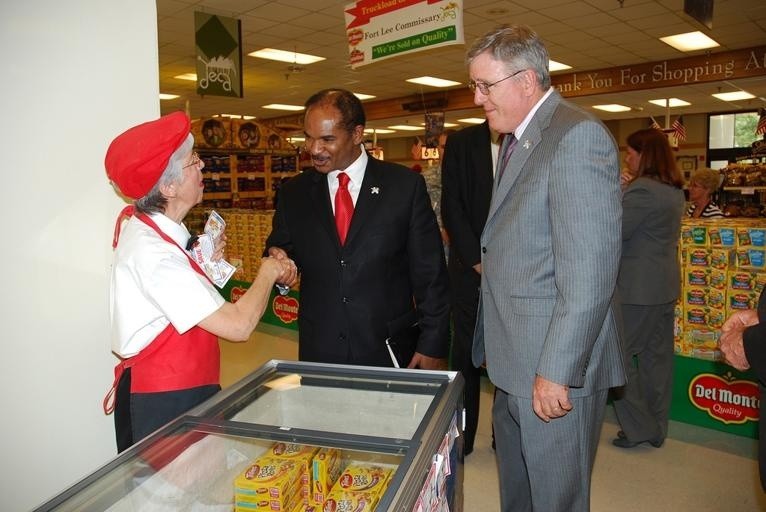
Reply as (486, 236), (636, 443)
(674, 216), (766, 363)
(205, 209), (301, 292)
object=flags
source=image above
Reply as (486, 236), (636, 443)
(646, 114), (661, 134)
(343, 0), (466, 70)
(412, 135), (423, 159)
(193, 12), (243, 98)
(672, 114), (689, 143)
(755, 108), (766, 135)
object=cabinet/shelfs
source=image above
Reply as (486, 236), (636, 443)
(192, 146), (302, 208)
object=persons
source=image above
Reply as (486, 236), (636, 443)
(102, 112), (296, 454)
(264, 90), (448, 392)
(441, 116), (512, 455)
(689, 167), (727, 217)
(717, 278), (766, 497)
(213, 125), (217, 135)
(466, 23), (629, 512)
(240, 129), (258, 147)
(208, 128), (212, 138)
(612, 125), (684, 448)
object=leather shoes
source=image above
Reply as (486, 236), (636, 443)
(613, 437), (664, 448)
(617, 430), (626, 438)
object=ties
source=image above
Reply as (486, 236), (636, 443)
(500, 136), (518, 180)
(335, 173), (354, 246)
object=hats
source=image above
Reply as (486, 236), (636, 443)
(105, 112), (191, 200)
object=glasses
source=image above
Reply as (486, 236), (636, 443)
(183, 152), (200, 170)
(468, 70), (522, 95)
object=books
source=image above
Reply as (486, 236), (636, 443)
(385, 321), (424, 371)
